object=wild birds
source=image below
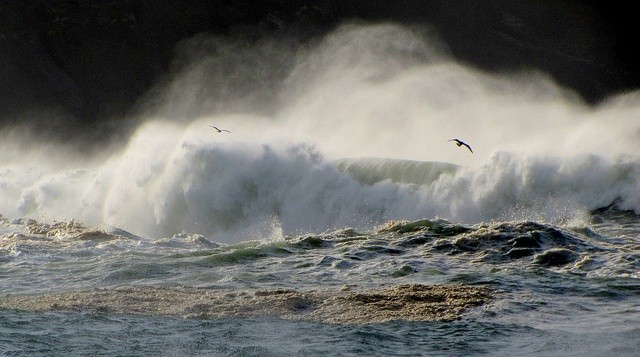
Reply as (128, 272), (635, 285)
(447, 137), (474, 155)
(208, 124), (232, 135)
